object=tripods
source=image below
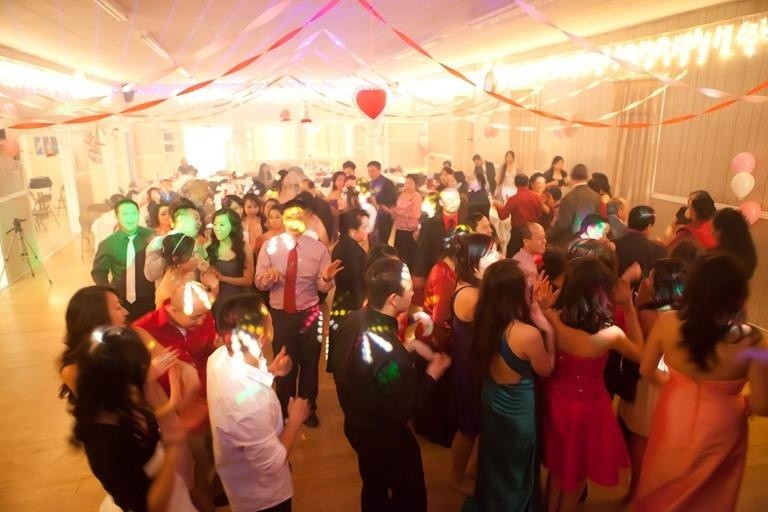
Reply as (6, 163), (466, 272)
(0, 229), (52, 285)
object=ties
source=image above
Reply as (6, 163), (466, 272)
(448, 219), (454, 231)
(125, 235), (137, 305)
(283, 241), (299, 315)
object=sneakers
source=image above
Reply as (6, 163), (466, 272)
(304, 414), (319, 427)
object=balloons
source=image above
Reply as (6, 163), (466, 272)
(356, 90), (385, 120)
(3, 139), (20, 158)
(740, 203), (762, 227)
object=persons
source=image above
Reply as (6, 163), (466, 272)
(541, 257), (643, 511)
(206, 296), (309, 511)
(88, 149), (757, 429)
(624, 256), (767, 511)
(448, 233), (501, 495)
(126, 280), (218, 510)
(334, 259), (454, 511)
(54, 284), (178, 455)
(458, 259), (556, 511)
(71, 325), (195, 511)
(578, 258), (700, 504)
